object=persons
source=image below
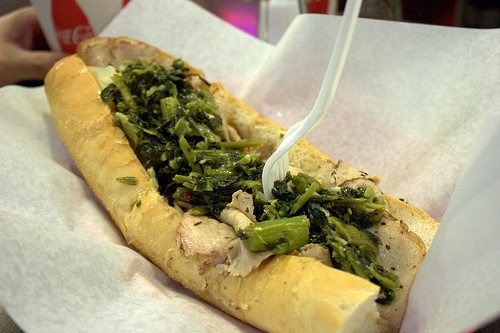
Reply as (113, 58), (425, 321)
(0, 4), (72, 87)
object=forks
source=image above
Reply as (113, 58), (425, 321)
(262, 0), (362, 200)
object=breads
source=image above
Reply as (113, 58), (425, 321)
(44, 37), (440, 333)
